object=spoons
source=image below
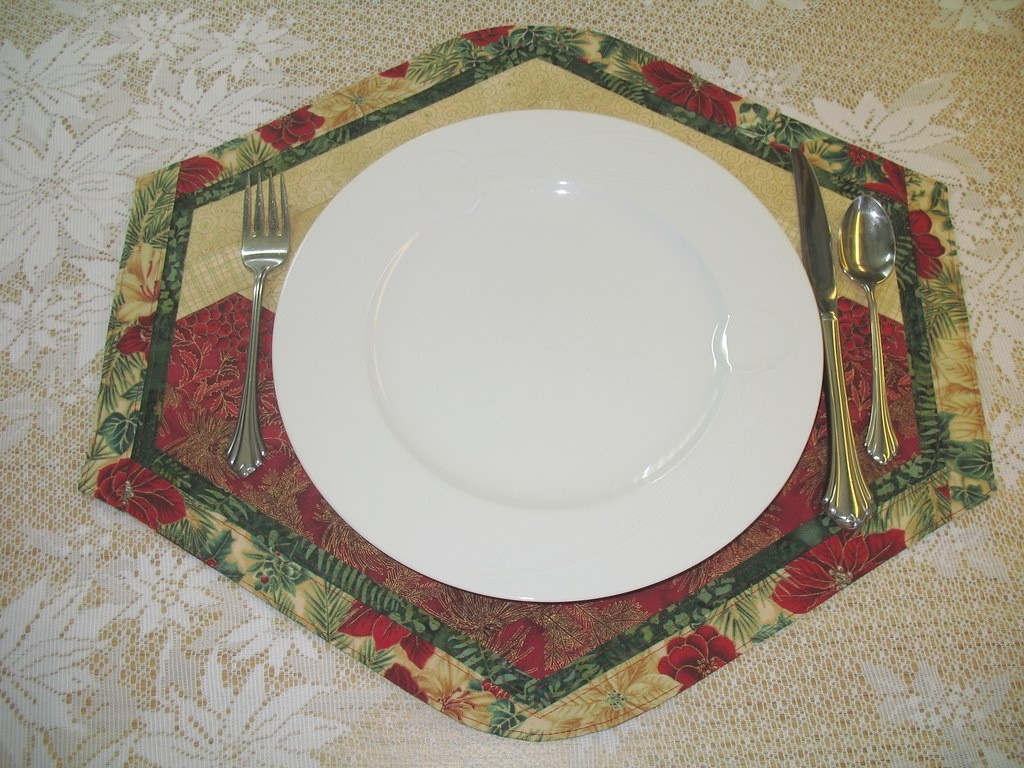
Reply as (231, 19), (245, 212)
(838, 194), (900, 463)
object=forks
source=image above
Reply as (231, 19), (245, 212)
(225, 171), (292, 476)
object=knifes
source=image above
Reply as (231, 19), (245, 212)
(790, 146), (873, 534)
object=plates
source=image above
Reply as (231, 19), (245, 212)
(272, 108), (825, 602)
